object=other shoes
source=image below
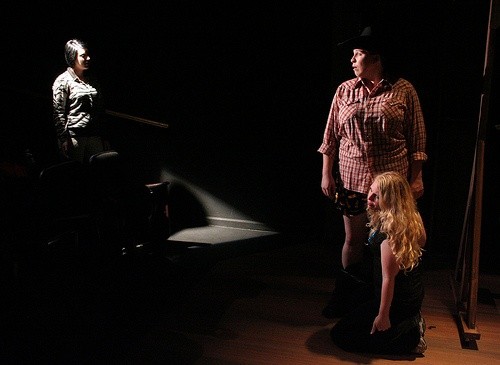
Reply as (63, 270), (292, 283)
(321, 301), (334, 318)
(415, 318), (428, 354)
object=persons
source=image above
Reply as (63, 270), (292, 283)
(317, 27), (428, 319)
(330, 170), (427, 354)
(52, 39), (112, 166)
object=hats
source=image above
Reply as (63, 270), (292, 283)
(336, 26), (398, 55)
